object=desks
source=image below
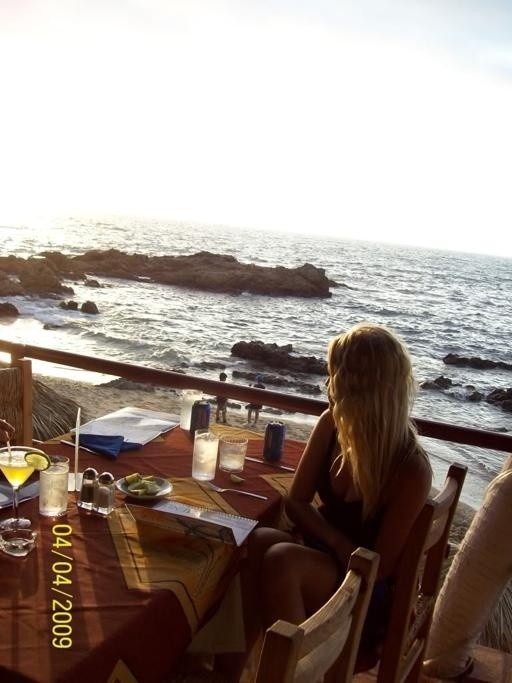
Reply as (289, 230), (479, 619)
(1, 405), (317, 683)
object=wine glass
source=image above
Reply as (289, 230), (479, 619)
(0, 445), (46, 531)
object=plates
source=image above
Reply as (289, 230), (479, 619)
(115, 476), (173, 501)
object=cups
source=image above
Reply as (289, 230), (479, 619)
(219, 434), (248, 474)
(191, 428), (220, 482)
(179, 389), (204, 431)
(37, 454), (71, 519)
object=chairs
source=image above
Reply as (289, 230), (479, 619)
(370, 461), (469, 682)
(0, 352), (33, 451)
(242, 547), (382, 682)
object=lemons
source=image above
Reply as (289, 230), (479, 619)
(23, 451), (50, 472)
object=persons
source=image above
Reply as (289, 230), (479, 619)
(242, 326), (434, 673)
(216, 373), (233, 427)
(247, 376), (265, 426)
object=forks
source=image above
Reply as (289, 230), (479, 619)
(206, 482), (268, 501)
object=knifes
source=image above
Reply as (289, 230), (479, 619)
(244, 456), (295, 472)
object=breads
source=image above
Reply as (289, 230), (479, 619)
(124, 473), (161, 496)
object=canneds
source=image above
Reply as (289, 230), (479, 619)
(262, 420), (285, 460)
(190, 400), (211, 436)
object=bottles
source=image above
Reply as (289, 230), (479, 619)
(76, 468), (114, 518)
